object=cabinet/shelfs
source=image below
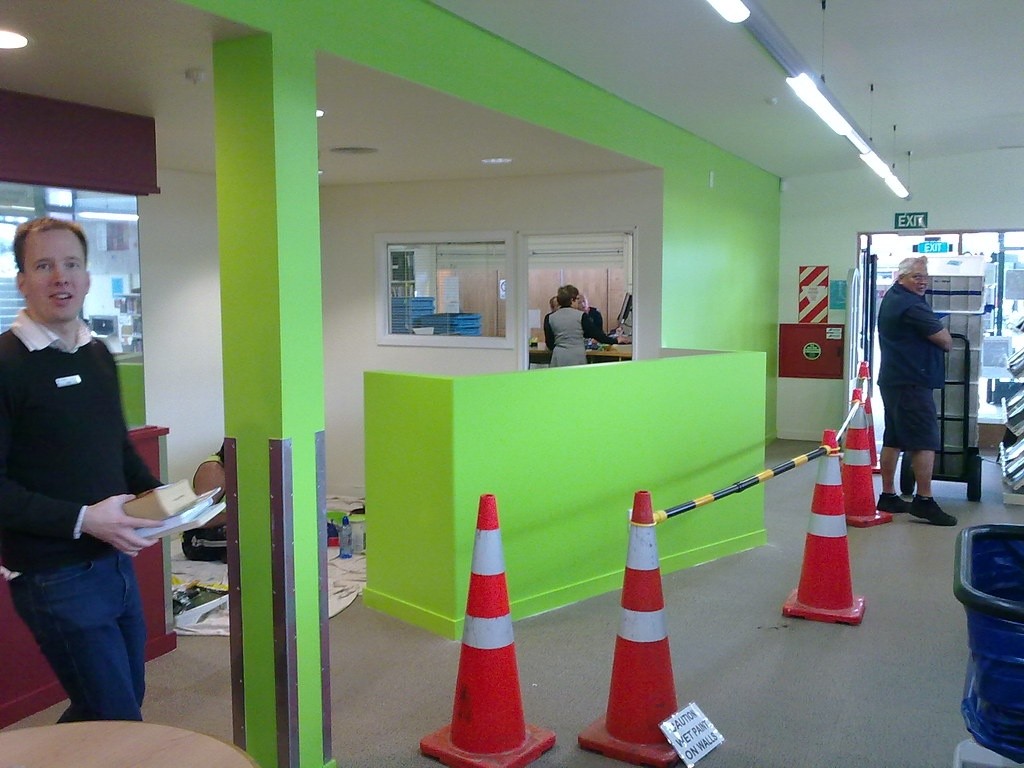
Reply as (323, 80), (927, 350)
(996, 310), (1024, 506)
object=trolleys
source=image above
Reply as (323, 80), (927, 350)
(900, 332), (983, 502)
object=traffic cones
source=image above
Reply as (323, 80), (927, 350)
(577, 490), (681, 768)
(856, 361), (881, 474)
(782, 429), (867, 625)
(420, 493), (557, 768)
(841, 388), (894, 527)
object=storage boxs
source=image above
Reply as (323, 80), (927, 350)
(953, 523), (1024, 768)
(391, 297), (482, 337)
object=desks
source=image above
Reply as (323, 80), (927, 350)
(529, 342), (632, 369)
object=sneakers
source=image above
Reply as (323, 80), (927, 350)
(877, 495), (911, 513)
(909, 494), (957, 526)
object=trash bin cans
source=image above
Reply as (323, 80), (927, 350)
(952, 522), (1024, 763)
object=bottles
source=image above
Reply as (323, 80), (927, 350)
(340, 516), (352, 559)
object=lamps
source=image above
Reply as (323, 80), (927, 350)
(707, 0), (914, 201)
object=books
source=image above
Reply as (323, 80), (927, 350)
(122, 478), (226, 542)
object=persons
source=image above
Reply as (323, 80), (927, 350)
(550, 296), (562, 313)
(543, 285), (631, 369)
(180, 437), (229, 562)
(0, 216), (164, 725)
(878, 257), (960, 526)
(577, 293), (602, 343)
